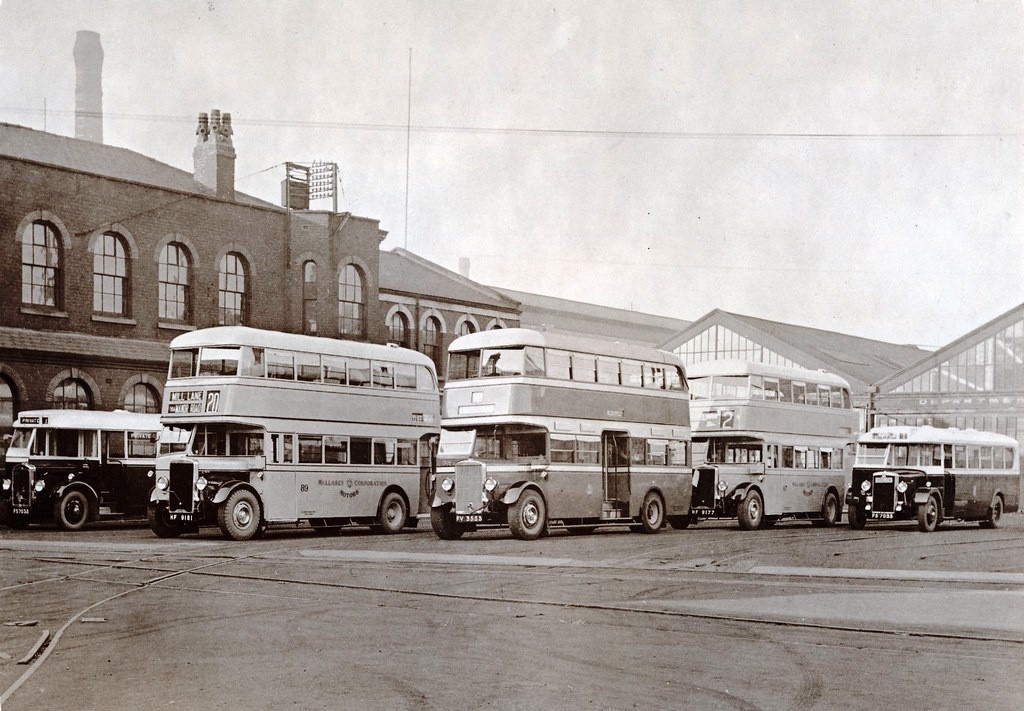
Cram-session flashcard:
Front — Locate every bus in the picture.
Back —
[145,326,441,541]
[668,360,859,530]
[845,426,1020,532]
[429,328,697,540]
[1,409,167,531]
